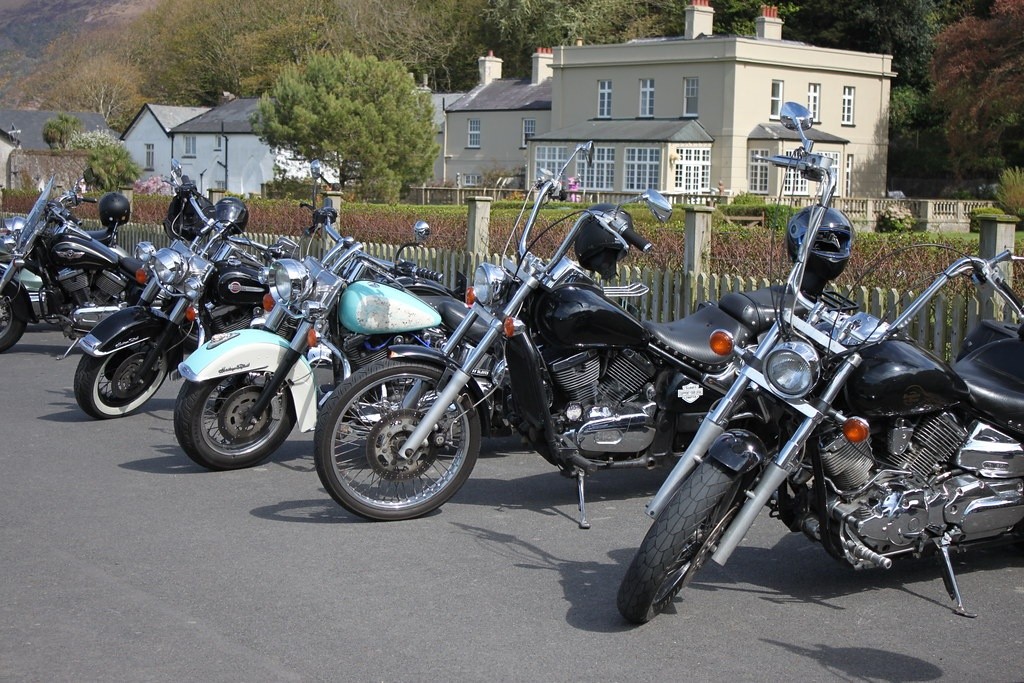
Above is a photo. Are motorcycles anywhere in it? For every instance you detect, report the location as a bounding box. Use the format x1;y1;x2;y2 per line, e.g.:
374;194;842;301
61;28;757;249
1;141;809;526
617;102;1024;627
